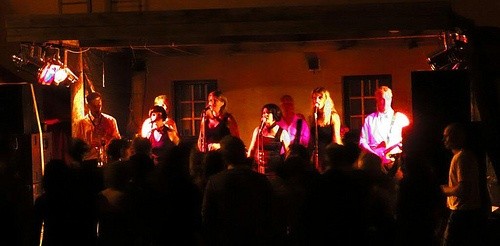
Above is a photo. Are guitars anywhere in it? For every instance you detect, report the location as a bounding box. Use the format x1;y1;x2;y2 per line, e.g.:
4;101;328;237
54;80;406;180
368;138;404;166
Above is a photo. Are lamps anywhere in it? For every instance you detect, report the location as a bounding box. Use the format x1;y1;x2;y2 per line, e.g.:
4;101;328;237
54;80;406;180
9;41;79;89
429;32;482;72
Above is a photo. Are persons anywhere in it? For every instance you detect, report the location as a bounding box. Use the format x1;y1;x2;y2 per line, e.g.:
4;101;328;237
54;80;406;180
438;122;487;246
72;92;121;164
199;134;275;246
247;104;291;176
307;88;344;145
358;86;411;162
197;88;239;153
33;86;500;246
140;95;179;146
270;142;316;190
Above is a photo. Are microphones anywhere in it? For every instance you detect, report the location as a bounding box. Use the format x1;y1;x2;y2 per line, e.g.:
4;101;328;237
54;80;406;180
202;105;211;113
258;118;266;132
314;103;319;117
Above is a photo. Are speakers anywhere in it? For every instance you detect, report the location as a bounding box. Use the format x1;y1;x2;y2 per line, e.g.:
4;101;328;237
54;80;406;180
410;70;471;123
16;132;53;184
19;182;44;204
